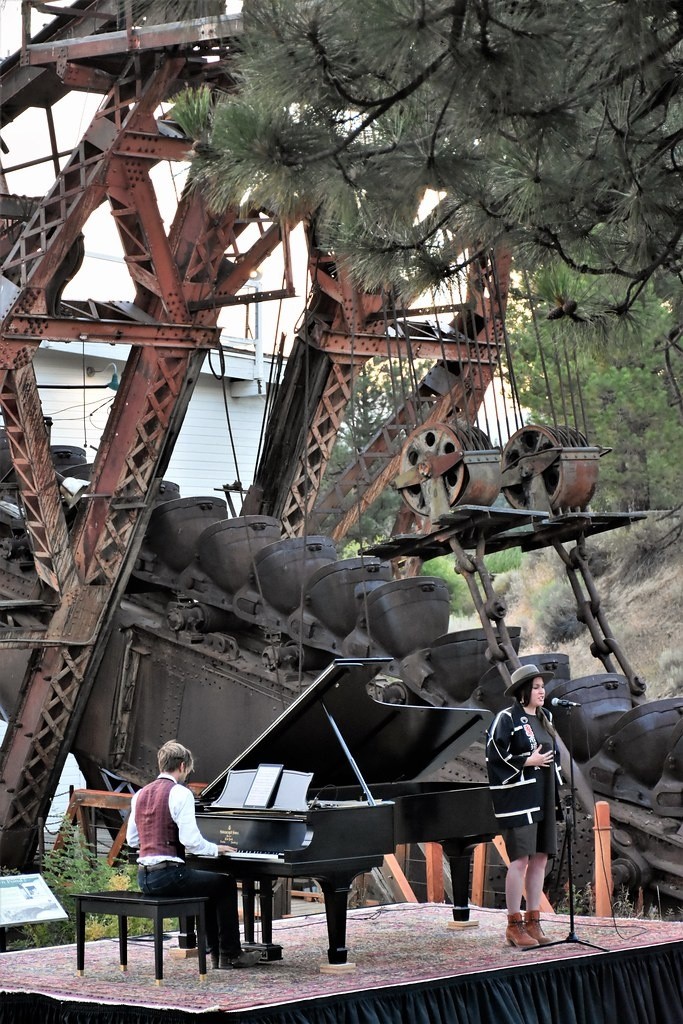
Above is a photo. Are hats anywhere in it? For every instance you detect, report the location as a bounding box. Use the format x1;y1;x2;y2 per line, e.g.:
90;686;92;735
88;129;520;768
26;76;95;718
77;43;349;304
504;664;555;697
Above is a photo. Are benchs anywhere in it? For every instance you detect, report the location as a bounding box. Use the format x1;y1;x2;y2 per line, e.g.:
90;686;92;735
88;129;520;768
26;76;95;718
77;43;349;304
69;889;208;987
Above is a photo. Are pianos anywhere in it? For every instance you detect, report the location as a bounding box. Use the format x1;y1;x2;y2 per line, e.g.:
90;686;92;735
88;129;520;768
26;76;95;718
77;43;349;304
165;659;497;982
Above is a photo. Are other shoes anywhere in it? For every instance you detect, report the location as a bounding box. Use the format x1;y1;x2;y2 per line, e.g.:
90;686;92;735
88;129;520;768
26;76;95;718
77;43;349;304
209;954;219;969
219;951;261;969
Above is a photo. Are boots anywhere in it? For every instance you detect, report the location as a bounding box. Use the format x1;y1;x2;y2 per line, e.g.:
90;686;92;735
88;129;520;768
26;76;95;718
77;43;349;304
505;912;540;948
524;910;552;944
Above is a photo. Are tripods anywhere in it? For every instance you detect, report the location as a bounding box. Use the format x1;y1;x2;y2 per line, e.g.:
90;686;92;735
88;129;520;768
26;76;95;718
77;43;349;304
521;707;610;953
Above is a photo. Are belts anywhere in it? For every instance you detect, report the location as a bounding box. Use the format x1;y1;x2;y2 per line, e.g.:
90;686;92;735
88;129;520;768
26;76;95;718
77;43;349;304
138;862;178;873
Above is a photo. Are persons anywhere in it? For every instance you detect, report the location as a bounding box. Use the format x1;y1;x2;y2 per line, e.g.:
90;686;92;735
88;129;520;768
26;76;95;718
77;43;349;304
126;738;262;969
486;664;564;948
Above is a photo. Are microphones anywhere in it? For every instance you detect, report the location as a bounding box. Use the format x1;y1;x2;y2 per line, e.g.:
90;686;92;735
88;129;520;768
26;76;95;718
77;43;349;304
551;698;582;708
